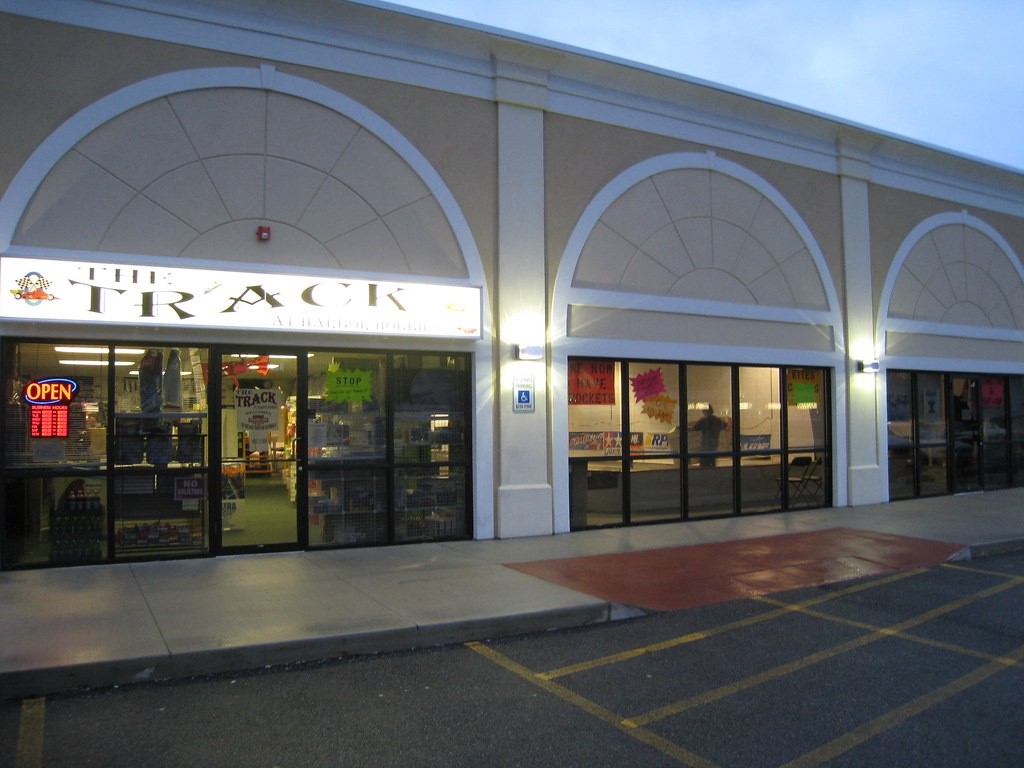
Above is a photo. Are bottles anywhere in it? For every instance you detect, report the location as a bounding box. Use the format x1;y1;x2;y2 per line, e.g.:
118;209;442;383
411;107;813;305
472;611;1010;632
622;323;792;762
49;488;105;547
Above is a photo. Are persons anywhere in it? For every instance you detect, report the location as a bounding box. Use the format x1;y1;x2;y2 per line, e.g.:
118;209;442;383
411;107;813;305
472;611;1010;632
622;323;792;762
676;403;728;468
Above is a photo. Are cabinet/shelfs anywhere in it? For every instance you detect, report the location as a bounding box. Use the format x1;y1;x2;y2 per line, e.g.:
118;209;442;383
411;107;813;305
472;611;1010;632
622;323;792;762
392;406;470;540
307;400;388;544
111;411;208;555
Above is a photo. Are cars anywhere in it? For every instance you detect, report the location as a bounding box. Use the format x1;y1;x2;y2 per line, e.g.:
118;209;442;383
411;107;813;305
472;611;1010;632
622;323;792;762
888;421;1005;471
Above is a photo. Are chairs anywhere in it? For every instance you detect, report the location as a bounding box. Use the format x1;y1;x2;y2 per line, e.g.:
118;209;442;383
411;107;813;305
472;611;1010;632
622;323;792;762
771;456;824;509
